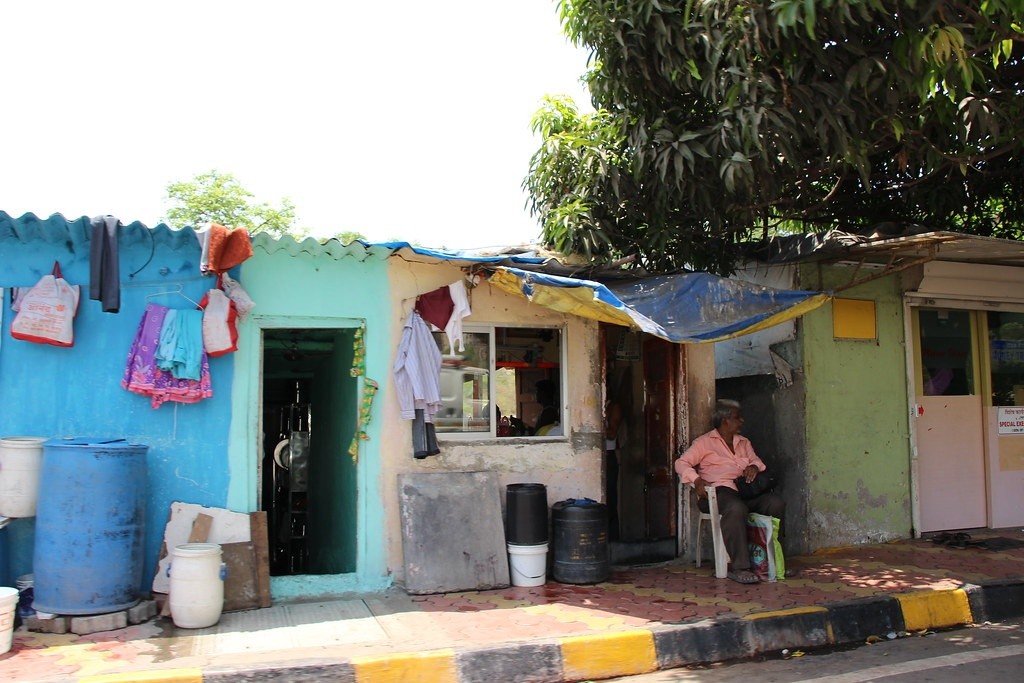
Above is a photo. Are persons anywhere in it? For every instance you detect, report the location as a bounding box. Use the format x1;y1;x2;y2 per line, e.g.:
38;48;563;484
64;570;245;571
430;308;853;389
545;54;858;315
674;398;798;584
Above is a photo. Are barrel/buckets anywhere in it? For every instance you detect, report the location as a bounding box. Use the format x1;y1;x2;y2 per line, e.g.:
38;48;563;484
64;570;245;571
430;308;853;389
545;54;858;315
552;500;610;584
33;435;150;615
16;573;36;618
507;543;548;586
166;543;224;628
506;483;548;545
0;587;20;654
0;437;45;517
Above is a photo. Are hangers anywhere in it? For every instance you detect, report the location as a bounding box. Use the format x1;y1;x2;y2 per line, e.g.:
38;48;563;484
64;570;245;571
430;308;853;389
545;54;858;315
141;282;205;317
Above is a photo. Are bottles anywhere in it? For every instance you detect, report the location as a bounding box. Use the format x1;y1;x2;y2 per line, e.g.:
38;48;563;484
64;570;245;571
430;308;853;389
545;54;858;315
502;416;509;436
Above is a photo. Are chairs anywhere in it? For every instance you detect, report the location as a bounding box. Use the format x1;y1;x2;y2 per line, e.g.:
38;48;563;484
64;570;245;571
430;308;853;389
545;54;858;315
688;482;778;583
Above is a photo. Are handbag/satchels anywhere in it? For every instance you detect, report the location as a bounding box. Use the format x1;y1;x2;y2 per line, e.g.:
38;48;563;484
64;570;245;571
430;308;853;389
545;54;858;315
747;513;786;583
733;471;775;501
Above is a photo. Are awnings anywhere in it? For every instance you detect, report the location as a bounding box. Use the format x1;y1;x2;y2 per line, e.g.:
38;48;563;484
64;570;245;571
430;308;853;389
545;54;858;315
470;261;835;346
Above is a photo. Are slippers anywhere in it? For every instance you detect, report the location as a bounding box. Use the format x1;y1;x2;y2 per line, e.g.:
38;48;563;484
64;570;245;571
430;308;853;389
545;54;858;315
728;569;759;584
785;569;795;578
